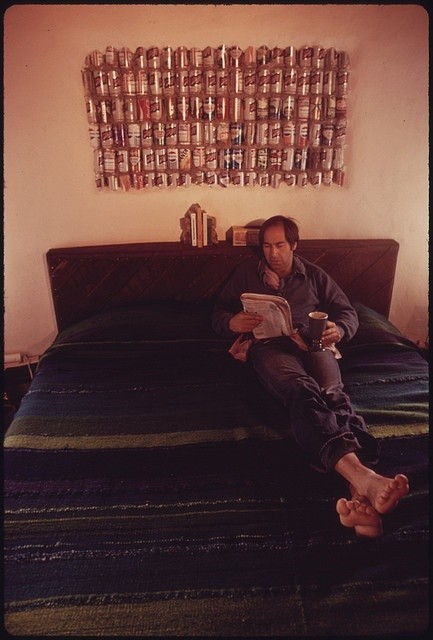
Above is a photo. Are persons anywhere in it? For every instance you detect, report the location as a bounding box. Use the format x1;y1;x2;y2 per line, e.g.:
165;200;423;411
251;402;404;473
209;216;411;539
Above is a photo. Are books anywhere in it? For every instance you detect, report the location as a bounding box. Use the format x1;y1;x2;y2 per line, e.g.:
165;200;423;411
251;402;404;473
240;293;293;339
183;203;219;248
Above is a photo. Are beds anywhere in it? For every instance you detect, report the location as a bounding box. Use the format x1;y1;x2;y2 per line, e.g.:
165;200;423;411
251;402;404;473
0;241;429;635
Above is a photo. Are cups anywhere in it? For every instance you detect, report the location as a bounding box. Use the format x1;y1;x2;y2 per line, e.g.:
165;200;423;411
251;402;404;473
308;312;328;342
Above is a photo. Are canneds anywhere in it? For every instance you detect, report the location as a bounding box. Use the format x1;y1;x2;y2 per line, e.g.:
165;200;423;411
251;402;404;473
311;45;325;69
217;122;229;144
244;172;257;188
230;45;244;67
119;175;131;191
324;47;338;68
206;148;218;170
156;173;167;189
138;99;151;122
133;174;143;190
202;46;217;67
284;46;297;67
103;149;116;173
147;46;160;69
270;174;284;189
153;123;166;145
188;69;203;95
243;98;256;120
309;124;321;147
310;68;323;94
144;173;155;190
218;147;231;171
334;126;346;146
82;70;95;96
216;98;229;120
179;124;191;145
191;122;204;145
310;97;322;121
175;46;189;69
105;175;114;190
124;99;137;122
268;122;281;144
112;124;126;148
190;47;203;68
281;148;294;171
244;69;258;95
322;126;334;146
175;70;189;95
122;71;136;96
337;69;348;92
271;69;283;93
257;45;271;67
323;171;334;187
256;69;270;93
323;95;336;119
111;98;125;123
91;50;105;68
180;173;192;188
195;171;205;186
117;150;128;173
203;70;216;94
332;170;344;187
283;122;295;146
243;46;257;67
167;148;179;169
271;46;284;67
107;70;122;97
230;122;242;146
127;124;140;148
149;70;163;95
94;175;103;191
115;177;122;190
298;46;312;67
294;148;308;171
129;150;141;173
179;149;191;169
297;69;310;95
190;97;203;119
243;149;256;171
269;97;283;120
118;47;132;68
168;172;180;189
232;149;243;170
136;70;148;96
321;148;334;170
134;46;148;68
177;96;190;121
307;147;321;170
230;68;244;95
96;99;112;124
258;148;268;170
93;70;109;96
165;123;178;145
323;71;336;95
105;45;119;68
141;122;154;147
258;174;269;188
284;174;296;187
154;149;167;171
150;99;163;122
336;95;347;113
268;149;283;171
298;97;310;121
162;70;175;94
310;172;322;189
162;45;174;70
94;150;104;174
216;70;229;95
205;171;218;187
338;51;350;69
100;124;114;148
232;172;244;187
256;123;268;145
142;149;155;170
332;148;344;169
202;97;217;121
283;68;298;94
229;98;243;122
296;123;308;146
164;97;177;122
218;172;230;189
217;44;231;68
89;125;101;149
283;96;296;121
297;172;308;189
85;98;97;123
243;122;255;145
257;98;269;121
204;122;217;144
192;146;206;168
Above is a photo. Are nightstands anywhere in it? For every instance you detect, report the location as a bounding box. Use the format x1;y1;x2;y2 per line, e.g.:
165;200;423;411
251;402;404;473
2;361;37;433
